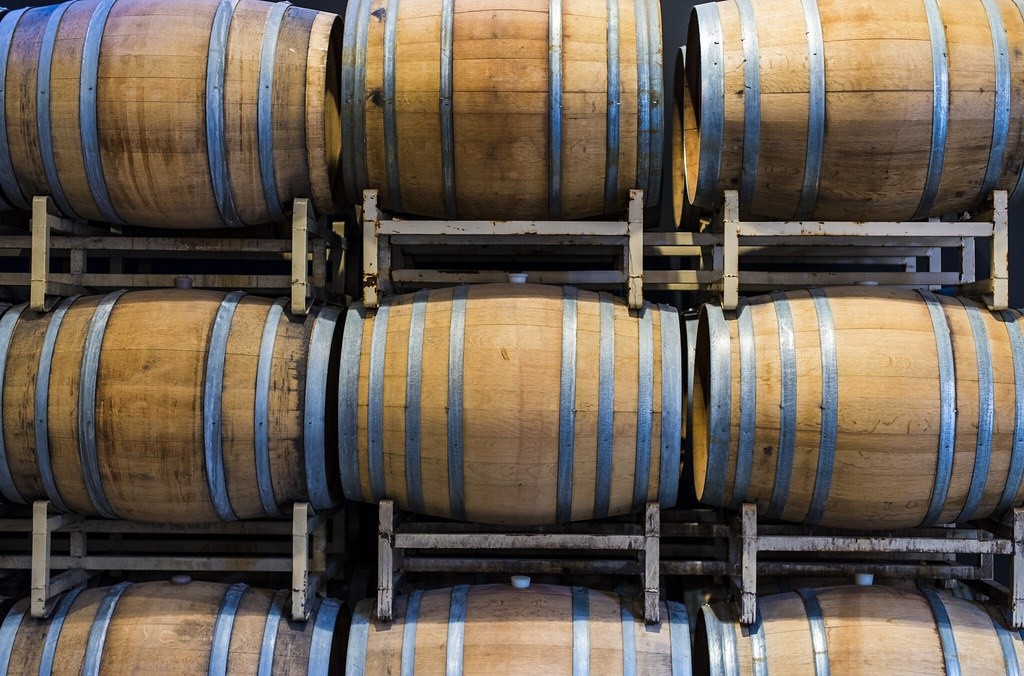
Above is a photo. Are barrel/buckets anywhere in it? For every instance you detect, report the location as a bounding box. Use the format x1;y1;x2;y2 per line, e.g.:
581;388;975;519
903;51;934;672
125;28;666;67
0;1;342;230
678;573;1024;676
1;575;346;676
345;574;693;676
338;271;679;525
343;1;663;229
671;1;1024;234
682;283;1024;530
0;277;344;523
